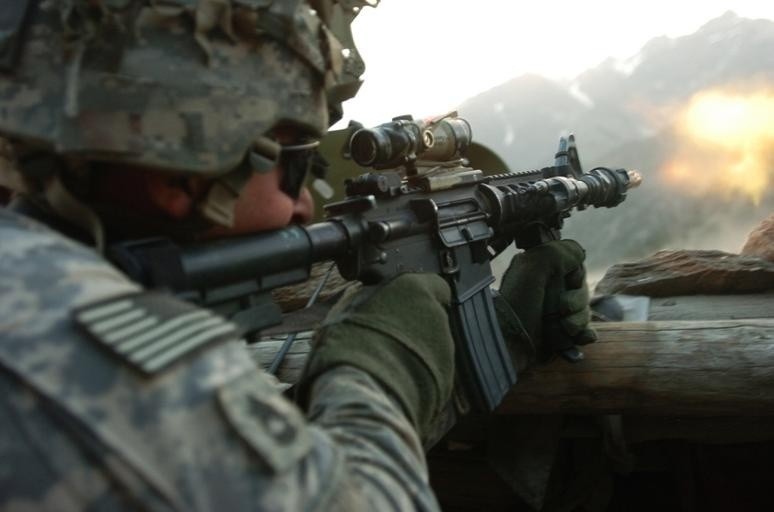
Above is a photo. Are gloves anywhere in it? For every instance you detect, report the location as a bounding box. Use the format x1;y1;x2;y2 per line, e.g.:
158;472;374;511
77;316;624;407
297;274;456;436
498;239;587;357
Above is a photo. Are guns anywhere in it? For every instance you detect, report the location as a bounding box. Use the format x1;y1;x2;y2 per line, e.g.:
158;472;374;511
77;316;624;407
103;112;640;414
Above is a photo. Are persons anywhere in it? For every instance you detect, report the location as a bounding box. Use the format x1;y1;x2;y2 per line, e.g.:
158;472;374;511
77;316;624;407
0;0;601;511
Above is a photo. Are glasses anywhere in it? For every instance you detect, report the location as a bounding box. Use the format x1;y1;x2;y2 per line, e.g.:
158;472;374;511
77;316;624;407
278;141;321;198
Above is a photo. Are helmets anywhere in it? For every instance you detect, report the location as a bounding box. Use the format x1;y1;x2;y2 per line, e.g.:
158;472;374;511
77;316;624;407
0;2;328;176
